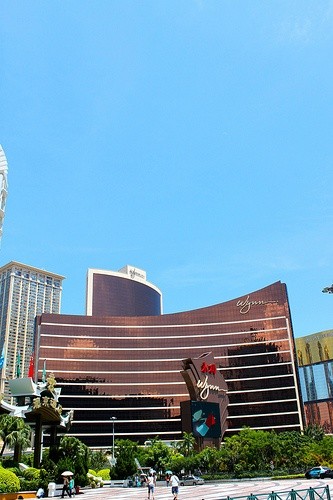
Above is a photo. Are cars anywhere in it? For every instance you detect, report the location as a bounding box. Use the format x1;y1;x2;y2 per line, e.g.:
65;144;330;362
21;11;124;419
305;466;333;480
178;476;204;486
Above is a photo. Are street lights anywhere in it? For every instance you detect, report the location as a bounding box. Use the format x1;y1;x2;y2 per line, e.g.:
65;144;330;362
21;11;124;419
110;416;117;464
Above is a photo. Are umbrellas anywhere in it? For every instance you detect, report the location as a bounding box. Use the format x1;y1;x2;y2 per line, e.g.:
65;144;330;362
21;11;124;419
61;471;74;476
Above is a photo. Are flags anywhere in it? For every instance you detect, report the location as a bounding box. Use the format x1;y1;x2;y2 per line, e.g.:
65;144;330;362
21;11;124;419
0;341;7;370
17;346;21;378
42;360;47;384
28;351;34;378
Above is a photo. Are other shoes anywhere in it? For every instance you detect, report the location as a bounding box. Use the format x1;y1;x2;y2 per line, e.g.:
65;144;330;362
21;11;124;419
173;497;176;500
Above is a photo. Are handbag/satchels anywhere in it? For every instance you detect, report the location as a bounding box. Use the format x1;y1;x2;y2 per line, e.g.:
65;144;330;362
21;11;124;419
71;488;76;493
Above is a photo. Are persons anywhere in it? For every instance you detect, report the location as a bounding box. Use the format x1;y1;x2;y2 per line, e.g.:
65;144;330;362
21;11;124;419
127;471;175;487
60;476;75;499
146;472;156;500
170;472;180;500
36;487;44;499
